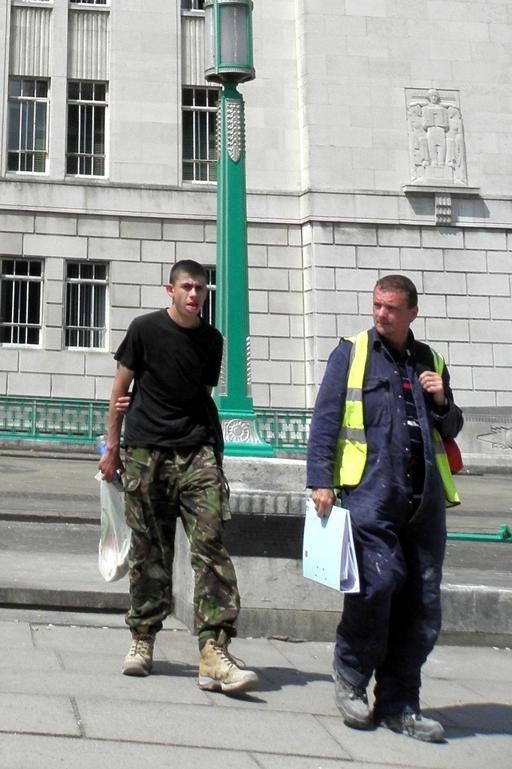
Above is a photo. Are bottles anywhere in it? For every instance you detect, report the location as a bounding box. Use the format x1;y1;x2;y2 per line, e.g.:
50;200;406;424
96;434;124;485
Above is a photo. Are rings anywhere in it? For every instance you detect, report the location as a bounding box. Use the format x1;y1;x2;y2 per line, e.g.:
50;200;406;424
430;381;433;386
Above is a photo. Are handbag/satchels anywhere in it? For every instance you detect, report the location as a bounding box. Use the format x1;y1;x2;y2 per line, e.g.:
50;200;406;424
94;468;133;583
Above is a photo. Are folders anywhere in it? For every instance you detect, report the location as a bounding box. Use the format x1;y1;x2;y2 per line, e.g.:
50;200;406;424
302;498;360;593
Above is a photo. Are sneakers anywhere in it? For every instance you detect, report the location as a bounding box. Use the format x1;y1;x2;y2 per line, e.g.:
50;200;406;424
331;659;371;728
373;700;446;742
199;629;258;694
122;625;157;676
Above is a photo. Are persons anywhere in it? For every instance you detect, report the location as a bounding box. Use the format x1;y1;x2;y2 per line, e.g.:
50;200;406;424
406;89;467;183
305;273;465;742
98;259;259;696
